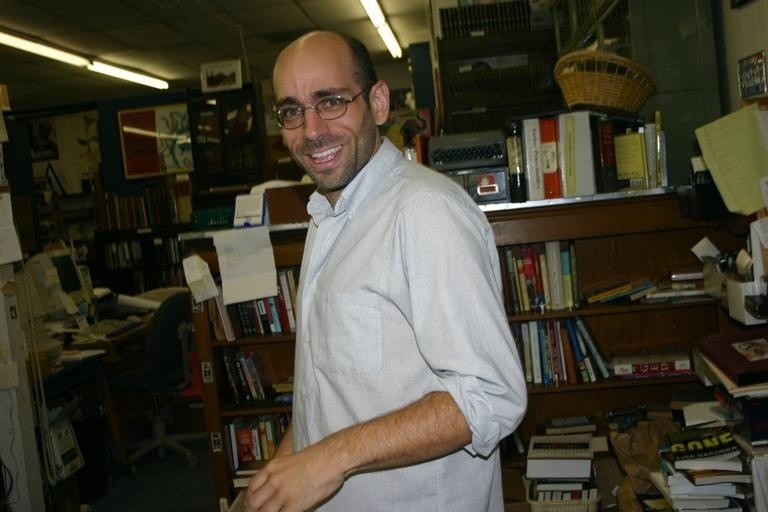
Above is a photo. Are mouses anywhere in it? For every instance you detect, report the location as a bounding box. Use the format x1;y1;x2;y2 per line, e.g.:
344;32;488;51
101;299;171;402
126;314;142;324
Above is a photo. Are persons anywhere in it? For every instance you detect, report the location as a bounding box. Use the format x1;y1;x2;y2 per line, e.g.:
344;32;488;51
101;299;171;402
242;30;532;510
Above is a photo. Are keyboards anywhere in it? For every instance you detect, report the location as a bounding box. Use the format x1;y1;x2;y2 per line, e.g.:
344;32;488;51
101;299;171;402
86;318;147;341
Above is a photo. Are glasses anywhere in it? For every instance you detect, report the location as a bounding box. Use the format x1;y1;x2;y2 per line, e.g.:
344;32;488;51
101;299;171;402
274;86;372;130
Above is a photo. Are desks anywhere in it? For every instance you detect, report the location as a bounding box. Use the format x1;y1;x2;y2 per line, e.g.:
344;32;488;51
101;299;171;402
29;310;154;480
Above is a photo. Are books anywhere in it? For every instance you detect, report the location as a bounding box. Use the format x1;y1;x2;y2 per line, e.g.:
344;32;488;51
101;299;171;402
502;111;647;203
503;240;581;313
513;415;609;510
604;389;768;510
206;262;300;508
33;183;174;294
582;264;705;304
508;316;611;388
606;345;695;380
694;330;768;448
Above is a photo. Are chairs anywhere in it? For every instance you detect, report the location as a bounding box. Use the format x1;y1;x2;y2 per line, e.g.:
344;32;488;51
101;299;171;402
105;286;208;476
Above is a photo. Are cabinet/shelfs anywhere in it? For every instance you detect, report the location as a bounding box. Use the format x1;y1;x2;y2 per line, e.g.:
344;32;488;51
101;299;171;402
1;83;268;295
177;184;768;511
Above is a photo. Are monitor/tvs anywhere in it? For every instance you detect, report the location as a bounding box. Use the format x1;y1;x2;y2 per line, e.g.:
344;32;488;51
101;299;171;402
21;247;89;317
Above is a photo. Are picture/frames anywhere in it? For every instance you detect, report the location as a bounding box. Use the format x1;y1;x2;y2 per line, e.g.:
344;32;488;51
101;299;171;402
735;50;768;102
198;58;242;95
